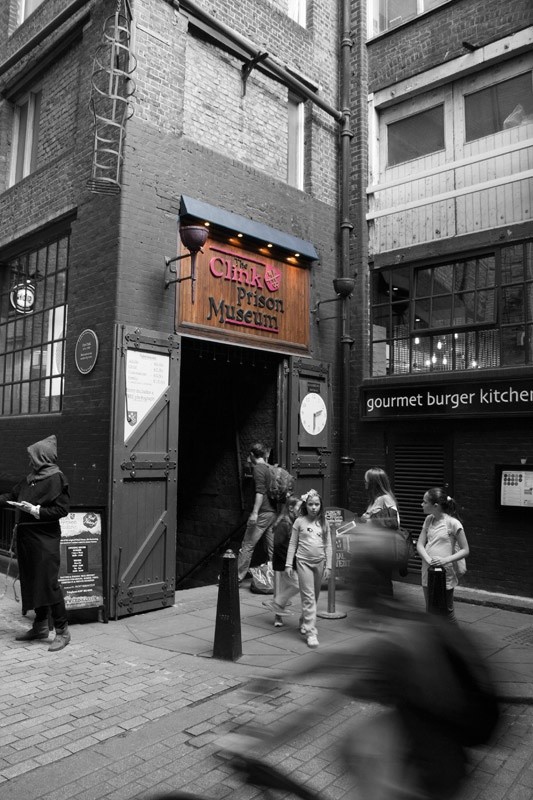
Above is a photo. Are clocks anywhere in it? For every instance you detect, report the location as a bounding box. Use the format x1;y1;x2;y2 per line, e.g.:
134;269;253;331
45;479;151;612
298;393;328;435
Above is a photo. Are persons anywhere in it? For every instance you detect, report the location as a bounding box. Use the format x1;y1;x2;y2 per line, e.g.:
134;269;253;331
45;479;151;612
0;434;72;652
284;489;332;649
272;498;303;627
416;487;470;625
217;443;278;588
359;467;400;528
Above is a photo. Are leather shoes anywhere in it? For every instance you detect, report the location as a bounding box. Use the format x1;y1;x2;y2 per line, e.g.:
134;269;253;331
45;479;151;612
16;627;48;639
49;631;71;650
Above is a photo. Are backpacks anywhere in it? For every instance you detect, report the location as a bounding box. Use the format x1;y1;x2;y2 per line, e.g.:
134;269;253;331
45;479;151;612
260;462;296;503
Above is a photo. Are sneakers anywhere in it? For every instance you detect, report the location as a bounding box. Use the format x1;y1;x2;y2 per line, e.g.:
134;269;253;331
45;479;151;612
309;634;319;646
275;615;283;626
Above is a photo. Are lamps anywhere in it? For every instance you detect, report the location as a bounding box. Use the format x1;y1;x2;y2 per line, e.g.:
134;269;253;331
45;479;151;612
318;277;357;337
164;226;209;304
